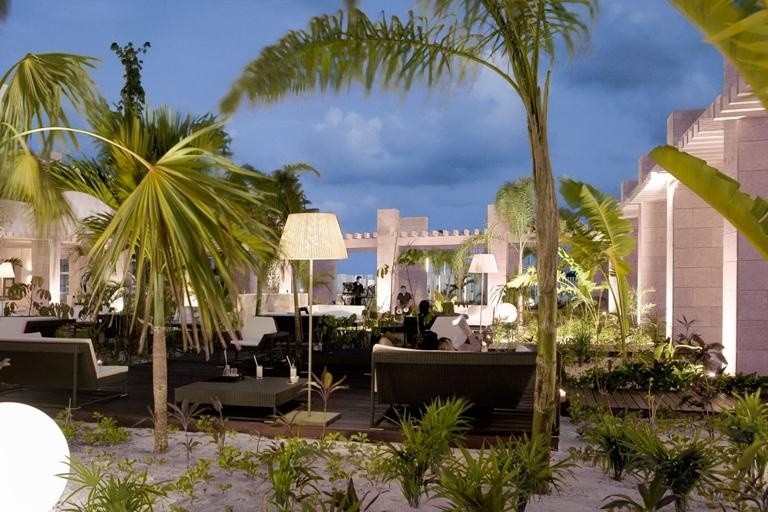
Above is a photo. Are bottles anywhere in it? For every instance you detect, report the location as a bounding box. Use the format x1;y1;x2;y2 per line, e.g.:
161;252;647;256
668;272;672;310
223;365;230;376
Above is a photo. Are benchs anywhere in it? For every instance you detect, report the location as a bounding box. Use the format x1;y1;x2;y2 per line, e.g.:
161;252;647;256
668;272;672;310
1;292;542;429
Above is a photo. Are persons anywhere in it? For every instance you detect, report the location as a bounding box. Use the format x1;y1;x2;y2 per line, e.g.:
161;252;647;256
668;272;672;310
397;285;413;314
353;276;365;304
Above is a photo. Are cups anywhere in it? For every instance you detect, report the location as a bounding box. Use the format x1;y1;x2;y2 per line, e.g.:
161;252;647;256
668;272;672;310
230;368;238;375
256;365;264;381
290;366;299;384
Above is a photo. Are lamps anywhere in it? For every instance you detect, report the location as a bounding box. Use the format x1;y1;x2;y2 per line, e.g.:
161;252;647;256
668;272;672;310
463;251;500;336
0;260;17;295
271;212;351;429
